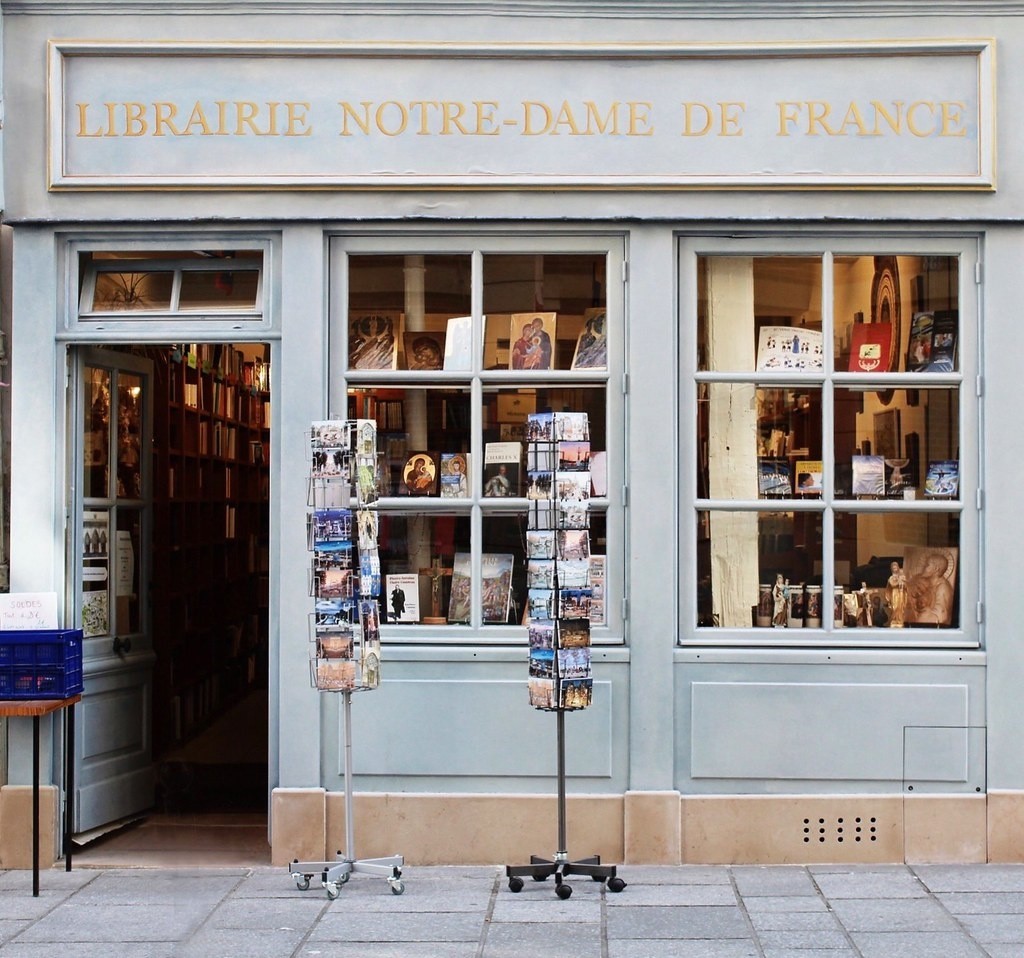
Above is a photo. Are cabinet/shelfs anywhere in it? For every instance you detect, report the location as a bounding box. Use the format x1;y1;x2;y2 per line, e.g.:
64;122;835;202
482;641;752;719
743;356;862;641
92;343;550;754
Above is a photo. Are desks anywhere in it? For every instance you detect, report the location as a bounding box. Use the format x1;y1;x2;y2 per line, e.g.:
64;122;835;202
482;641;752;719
0;690;87;900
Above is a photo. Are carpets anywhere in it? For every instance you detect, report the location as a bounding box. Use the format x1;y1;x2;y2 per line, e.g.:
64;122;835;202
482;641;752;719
153;760;269;814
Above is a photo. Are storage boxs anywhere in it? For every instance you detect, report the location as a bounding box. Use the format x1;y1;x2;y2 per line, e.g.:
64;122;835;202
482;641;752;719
0;629;84;701
0;782;63;876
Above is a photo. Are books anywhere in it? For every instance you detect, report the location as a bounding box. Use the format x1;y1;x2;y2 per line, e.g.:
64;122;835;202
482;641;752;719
852;456;959;497
528;411;593;708
849;322;892;372
508;313;557;369
168;343;272;740
767;430;809;457
759;461;823;494
349;396;408;556
448;552;514;623
571;308;607;370
697;364;709;538
908;309;958;365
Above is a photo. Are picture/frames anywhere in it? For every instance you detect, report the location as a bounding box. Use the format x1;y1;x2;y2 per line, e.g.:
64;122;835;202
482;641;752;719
568;308;607;371
445;548;515;625
396;450;444;500
506;312;555;375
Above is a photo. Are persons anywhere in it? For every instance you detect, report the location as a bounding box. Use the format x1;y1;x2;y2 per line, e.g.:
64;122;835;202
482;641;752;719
772;574;789;625
798;473;814;488
885;562;908;628
486;465;508;496
909;554;953;622
406;458;432;491
852;582;872;627
309;419;381;691
391;585;406;620
512;318;552;370
